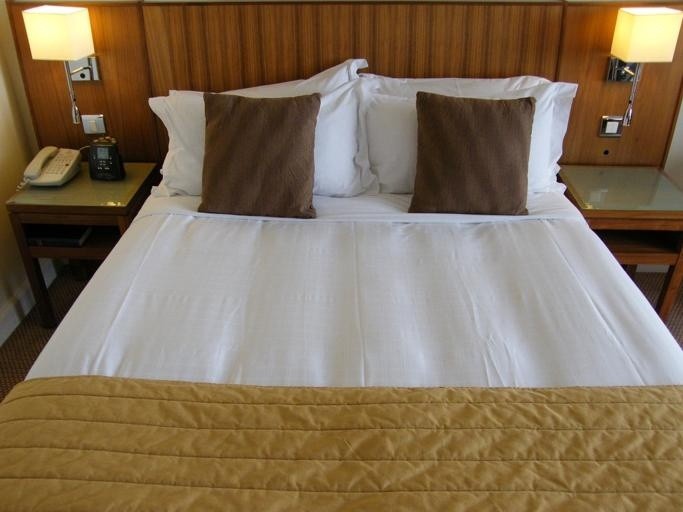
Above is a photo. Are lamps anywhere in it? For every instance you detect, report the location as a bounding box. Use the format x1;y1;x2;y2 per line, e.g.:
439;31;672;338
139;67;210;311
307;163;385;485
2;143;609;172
606;6;683;127
22;4;101;125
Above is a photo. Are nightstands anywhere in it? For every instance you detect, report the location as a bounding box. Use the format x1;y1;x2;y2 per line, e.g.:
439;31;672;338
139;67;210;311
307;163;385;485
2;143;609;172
6;162;159;331
557;164;683;323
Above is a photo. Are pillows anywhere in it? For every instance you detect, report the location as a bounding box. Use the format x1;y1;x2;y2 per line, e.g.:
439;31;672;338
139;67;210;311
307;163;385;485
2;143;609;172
149;77;377;198
365;82;579;198
359;72;551;97
407;91;535;216
169;59;369;99
197;92;321;219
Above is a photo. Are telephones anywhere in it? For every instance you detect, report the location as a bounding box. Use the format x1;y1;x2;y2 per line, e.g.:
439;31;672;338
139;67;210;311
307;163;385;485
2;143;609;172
23;146;81;186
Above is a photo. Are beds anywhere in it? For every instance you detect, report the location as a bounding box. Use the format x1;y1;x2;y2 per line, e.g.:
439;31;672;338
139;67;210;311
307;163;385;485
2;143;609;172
0;0;683;512
